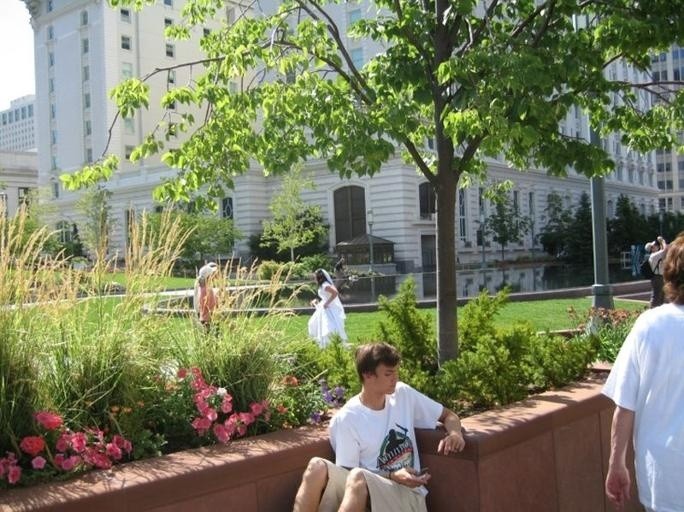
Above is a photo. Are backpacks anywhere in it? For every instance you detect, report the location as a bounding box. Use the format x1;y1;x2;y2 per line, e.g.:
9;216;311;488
639;257;661;280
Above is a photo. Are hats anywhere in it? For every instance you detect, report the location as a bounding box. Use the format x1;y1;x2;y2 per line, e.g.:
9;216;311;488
197;265;216;281
645;240;656;253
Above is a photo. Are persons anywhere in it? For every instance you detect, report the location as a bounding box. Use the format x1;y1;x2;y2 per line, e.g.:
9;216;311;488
198;266;218;331
644;236;668;309
292;341;465;512
307;269;348;350
334;258;346;278
193;262;217;319
601;235;684;512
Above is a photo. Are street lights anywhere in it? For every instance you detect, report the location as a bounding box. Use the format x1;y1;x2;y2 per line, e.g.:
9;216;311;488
367;209;376;303
480;214;487;290
659;212;664;236
571;9;625;339
